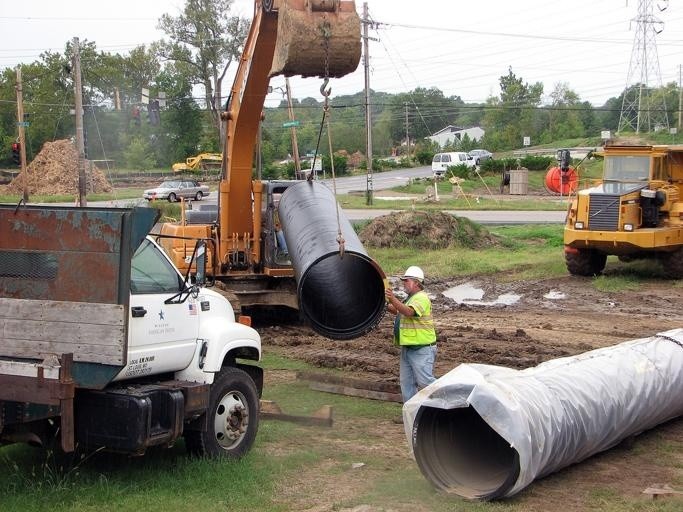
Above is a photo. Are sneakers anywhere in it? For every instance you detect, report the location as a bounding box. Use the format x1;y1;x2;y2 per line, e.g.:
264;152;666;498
394;416;403;423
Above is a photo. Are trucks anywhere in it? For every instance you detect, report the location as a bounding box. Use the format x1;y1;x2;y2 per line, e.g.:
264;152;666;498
1;194;268;471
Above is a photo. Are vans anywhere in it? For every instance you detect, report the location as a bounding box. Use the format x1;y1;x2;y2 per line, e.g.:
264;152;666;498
431;151;476;177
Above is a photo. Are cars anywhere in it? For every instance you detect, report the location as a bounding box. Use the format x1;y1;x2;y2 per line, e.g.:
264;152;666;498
141;178;210;203
468;148;493;165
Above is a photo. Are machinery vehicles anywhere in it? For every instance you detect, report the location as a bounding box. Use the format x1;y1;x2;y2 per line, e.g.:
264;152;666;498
562;140;682;279
145;0;363;332
171;150;224;175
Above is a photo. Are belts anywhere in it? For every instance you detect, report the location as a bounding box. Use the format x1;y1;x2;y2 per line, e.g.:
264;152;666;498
423;341;436;346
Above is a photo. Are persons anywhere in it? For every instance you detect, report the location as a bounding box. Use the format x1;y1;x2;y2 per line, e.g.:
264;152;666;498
384;265;438;424
270;204;288;258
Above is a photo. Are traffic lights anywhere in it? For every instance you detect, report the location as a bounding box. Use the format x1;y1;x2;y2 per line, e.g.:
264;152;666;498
12;143;19;165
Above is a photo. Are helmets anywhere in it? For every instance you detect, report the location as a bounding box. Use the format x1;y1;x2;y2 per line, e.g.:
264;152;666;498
400;266;425;282
